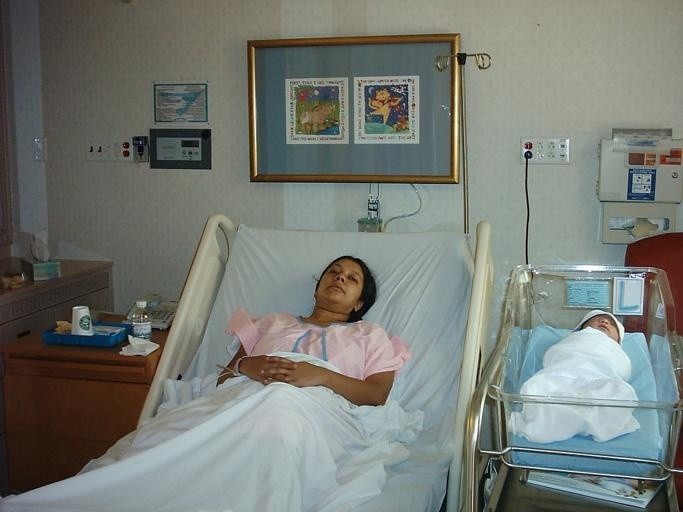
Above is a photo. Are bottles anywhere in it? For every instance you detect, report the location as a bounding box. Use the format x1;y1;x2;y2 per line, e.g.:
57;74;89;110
130;299;152;344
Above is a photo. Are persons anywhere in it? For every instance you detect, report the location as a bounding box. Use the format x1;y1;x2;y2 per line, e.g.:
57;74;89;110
511;309;640;445
215;255;396;406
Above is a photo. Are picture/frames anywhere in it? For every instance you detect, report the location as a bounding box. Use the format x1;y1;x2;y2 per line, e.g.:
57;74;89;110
247;33;463;184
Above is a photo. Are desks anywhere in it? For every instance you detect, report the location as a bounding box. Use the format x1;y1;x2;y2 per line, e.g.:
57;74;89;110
1;258;114;349
2;313;171;497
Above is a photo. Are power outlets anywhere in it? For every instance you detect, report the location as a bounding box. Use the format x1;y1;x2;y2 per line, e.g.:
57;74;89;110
519;136;570;166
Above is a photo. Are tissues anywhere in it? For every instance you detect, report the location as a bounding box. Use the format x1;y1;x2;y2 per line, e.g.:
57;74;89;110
19;240;62;281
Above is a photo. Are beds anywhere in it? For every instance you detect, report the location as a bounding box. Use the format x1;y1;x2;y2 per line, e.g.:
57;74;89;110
462;263;683;512
136;214;493;511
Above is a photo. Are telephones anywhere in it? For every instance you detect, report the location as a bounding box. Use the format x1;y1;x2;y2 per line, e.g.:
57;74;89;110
122;293;178;330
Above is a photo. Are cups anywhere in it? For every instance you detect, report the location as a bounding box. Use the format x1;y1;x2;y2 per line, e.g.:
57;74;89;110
70;304;93;337
